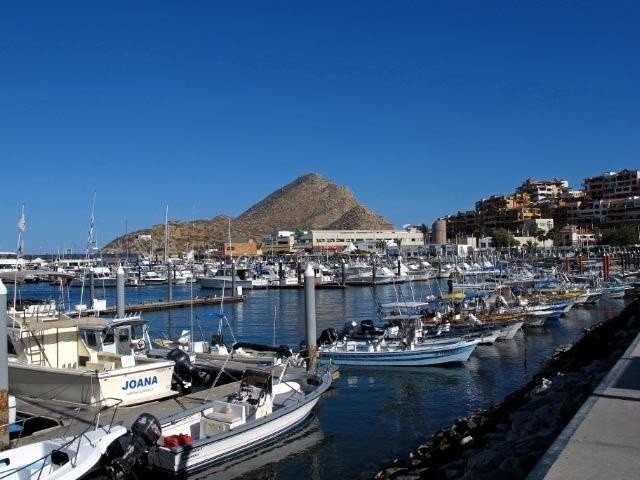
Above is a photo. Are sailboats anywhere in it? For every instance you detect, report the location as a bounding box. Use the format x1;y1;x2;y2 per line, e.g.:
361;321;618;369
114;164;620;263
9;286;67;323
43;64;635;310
612;299;624;306
381;257;523;338
557;317;577;330
514;332;525;345
564;309;592;323
525;327;554;343
470;344;501;359
494;338;520;357
338;365;473;389
419;260;525;340
574;250;613;308
544;318;568;335
613;250;640;298
340;252;502;344
497;259;545;326
300;252;483;365
152;243;307;368
545;250;574;317
463;356;481;372
71;190;196;366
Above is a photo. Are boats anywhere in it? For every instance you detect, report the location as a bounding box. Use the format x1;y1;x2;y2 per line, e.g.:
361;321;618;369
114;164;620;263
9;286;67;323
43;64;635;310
187;415;324;480
0;191;217;287
148;344;338;474
134;355;334;479
130;268;291;381
50;191;136;287
0;204;76;285
198;220;494;289
